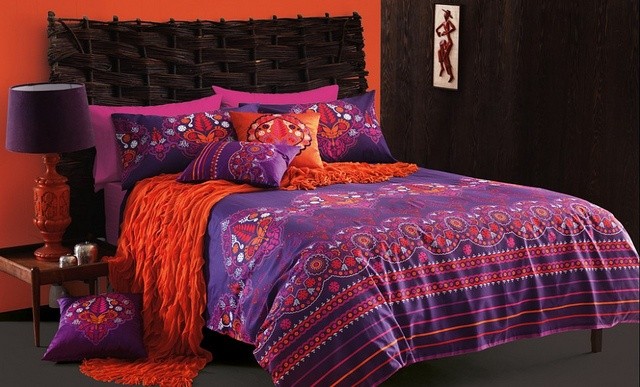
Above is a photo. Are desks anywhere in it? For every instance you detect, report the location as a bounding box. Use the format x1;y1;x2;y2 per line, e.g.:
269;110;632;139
1;240;118;348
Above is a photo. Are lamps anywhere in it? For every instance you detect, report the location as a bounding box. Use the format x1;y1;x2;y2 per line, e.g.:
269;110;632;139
6;79;98;263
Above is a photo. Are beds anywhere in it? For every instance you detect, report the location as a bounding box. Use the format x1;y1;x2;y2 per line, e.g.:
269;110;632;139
47;9;638;385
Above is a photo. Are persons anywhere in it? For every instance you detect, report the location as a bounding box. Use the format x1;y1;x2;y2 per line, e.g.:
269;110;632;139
435;7;456;82
438;39;446;76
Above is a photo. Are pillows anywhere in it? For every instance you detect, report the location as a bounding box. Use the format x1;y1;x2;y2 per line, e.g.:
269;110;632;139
210;82;339;105
109;107;240;194
85;91;226;194
228;107;326;170
238;87;398;165
40;291;150;361
177;138;302;186
